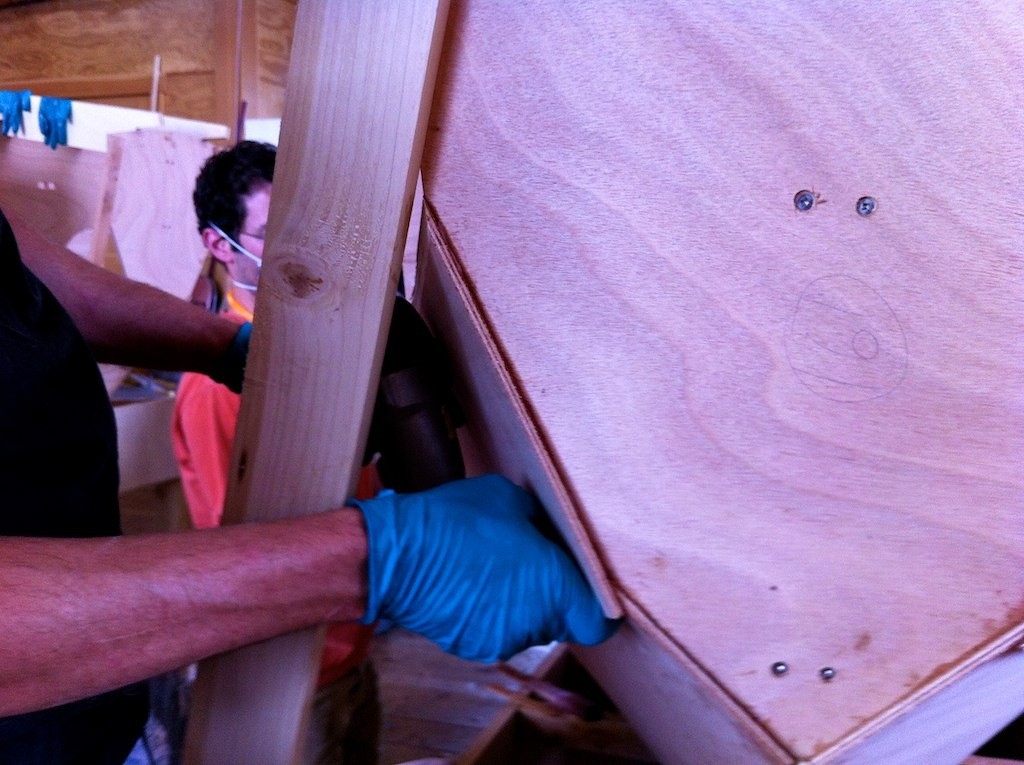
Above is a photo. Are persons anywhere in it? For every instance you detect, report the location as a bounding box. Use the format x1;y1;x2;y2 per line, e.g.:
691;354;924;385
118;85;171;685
167;138;384;765
0;207;623;765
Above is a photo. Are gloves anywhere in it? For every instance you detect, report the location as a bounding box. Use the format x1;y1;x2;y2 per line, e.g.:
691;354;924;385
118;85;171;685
342;475;618;666
